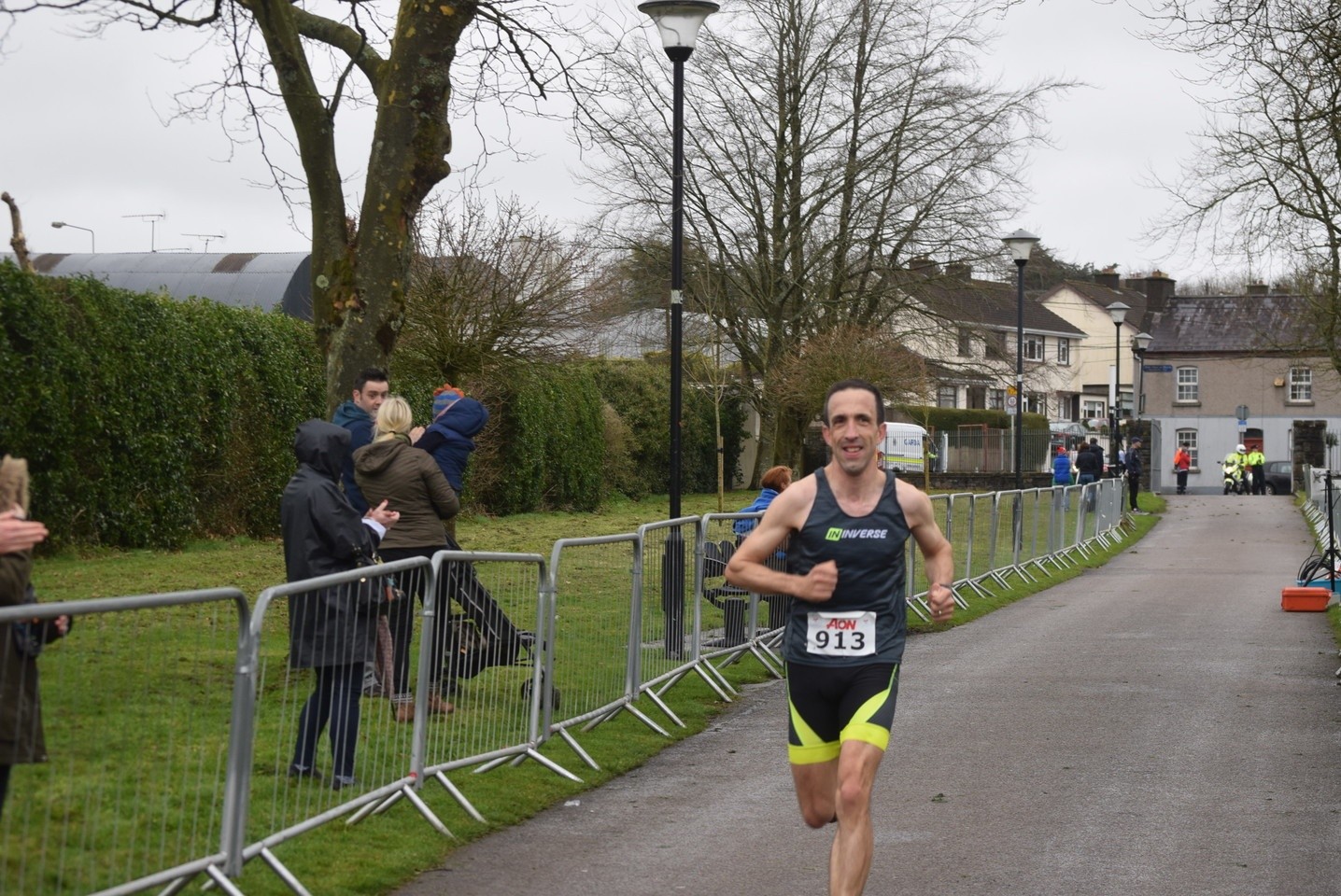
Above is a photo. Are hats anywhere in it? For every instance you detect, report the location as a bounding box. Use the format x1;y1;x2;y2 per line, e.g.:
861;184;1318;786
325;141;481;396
1131;437;1142;443
1058;447;1066;454
1090;438;1097;442
1251;444;1258;449
1183;442;1189;446
432;390;460;420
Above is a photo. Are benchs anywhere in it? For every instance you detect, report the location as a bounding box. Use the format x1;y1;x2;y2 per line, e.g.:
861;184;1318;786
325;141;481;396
703;540;772;647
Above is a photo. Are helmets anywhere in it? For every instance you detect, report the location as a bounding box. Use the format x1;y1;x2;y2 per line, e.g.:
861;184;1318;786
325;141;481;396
1236;444;1246;454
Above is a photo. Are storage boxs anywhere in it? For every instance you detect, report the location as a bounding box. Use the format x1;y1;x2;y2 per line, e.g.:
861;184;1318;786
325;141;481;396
1281;579;1341;610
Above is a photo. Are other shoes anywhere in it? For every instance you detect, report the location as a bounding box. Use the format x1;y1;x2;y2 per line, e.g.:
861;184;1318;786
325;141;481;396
430;679;453;691
428;695;453;715
394;687;412;698
289;763;325;780
1087;500;1091;512
364;683;387;697
394;703;415;722
333;777;358;790
1065;508;1068;511
1132;508;1150;515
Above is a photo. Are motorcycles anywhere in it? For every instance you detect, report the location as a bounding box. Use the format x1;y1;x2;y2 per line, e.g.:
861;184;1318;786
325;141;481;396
1216;458;1244;495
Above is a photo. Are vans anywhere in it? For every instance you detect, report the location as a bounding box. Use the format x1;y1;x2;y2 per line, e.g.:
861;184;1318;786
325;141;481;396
875;421;940;475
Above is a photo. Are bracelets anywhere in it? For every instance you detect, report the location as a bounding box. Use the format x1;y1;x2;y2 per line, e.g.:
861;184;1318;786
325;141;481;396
940;583;952;589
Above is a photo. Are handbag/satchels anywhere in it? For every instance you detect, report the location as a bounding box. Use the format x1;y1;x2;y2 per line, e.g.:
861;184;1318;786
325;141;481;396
355;555;407;616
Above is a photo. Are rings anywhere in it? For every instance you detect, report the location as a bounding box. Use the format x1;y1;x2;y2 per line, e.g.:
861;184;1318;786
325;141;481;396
938;610;942;616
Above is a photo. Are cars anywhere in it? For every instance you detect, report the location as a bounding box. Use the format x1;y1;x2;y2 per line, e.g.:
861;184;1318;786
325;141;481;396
1244;460;1292;495
1047;417;1109;454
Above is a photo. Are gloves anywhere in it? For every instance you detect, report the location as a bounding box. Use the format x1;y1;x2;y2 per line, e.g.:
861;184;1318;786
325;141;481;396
434;384;464;397
1174;464;1178;470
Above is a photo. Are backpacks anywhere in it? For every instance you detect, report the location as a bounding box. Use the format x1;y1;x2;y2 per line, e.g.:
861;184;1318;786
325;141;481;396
733;505;787;559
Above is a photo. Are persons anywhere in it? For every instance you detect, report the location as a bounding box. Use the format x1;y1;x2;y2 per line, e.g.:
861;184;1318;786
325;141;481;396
1053;446;1071;512
279;366;489;789
1126;435;1145;513
1248;444;1267;495
1229;444;1254;495
732;465;795;630
1075;442;1097;513
1089;437;1103;481
1173;442;1191;494
0;448;73;812
723;380;957;896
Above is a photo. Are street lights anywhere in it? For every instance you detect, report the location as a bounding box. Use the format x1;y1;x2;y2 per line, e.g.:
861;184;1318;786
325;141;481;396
1000;228;1041;550
1134;332;1153;416
1105;300;1131;478
50;221;97;253
637;0;725;664
